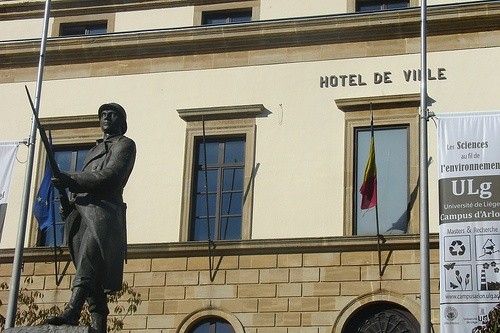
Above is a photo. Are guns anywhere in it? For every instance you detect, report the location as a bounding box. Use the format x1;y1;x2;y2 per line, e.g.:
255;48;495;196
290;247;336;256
25;84;72;219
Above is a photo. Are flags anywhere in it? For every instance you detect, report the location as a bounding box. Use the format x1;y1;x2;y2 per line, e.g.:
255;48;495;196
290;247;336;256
357;134;376;208
32;138;64;231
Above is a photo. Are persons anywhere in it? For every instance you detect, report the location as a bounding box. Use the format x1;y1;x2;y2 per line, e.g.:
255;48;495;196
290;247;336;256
44;103;136;333
